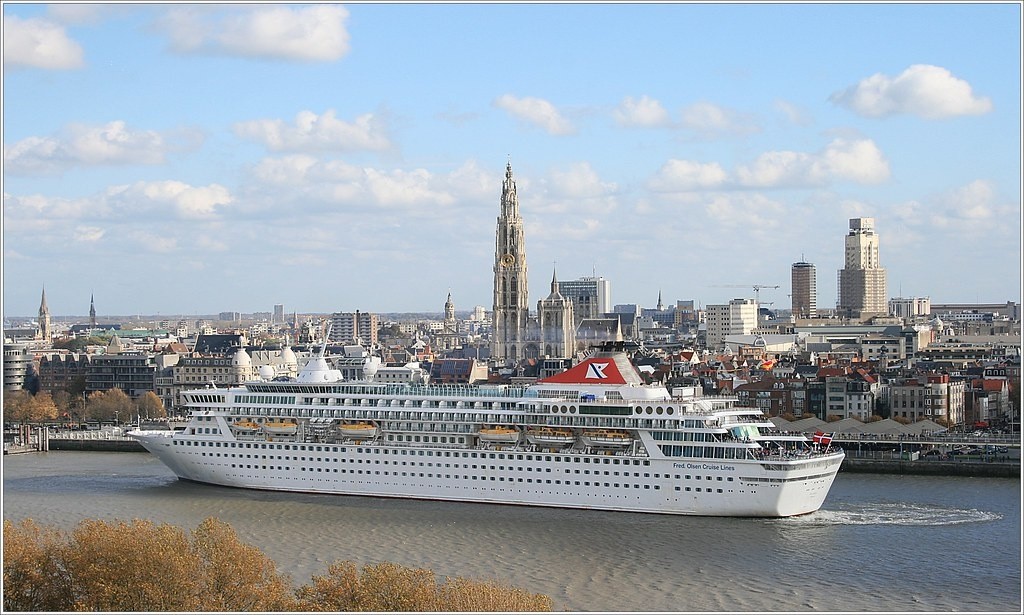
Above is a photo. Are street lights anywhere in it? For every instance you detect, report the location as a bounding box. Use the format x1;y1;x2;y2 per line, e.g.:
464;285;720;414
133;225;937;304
83;391;85;421
1008;402;1014;438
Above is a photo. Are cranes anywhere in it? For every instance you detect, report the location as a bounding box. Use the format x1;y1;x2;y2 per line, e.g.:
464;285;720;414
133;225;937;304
709;283;779;310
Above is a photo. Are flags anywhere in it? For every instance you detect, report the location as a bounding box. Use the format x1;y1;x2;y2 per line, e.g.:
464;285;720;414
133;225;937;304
813;431;833;445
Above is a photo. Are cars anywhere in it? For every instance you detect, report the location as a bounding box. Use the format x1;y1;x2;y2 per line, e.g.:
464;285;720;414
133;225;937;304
922;418;1010;459
4;423;78;432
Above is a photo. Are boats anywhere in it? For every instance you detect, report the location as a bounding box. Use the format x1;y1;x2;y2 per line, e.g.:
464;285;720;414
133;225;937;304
125;321;847;517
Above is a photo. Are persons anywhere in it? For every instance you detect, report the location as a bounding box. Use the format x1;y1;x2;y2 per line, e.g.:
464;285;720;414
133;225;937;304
724;434;751;444
772;428;806;436
838;431;916;441
755;445;842;457
